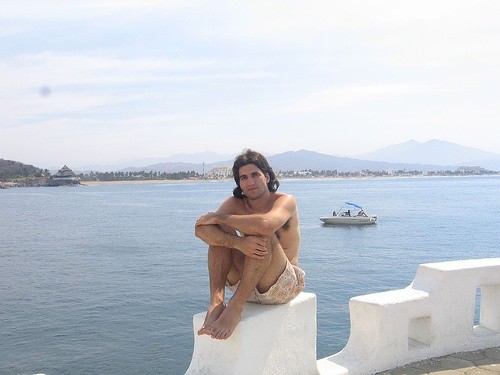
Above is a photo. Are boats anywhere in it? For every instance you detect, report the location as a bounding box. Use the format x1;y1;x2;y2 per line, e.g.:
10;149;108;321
318;200;377;225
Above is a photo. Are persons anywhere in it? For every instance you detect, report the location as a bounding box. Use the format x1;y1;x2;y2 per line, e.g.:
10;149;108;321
358;210;368;217
195;148;304;340
